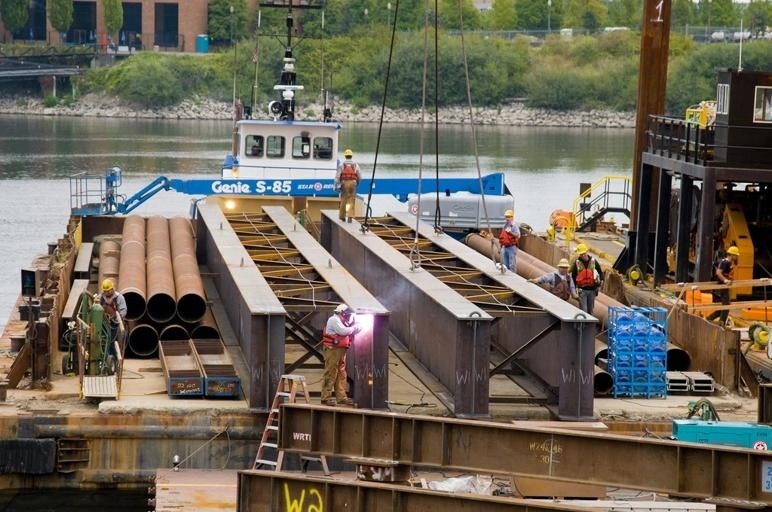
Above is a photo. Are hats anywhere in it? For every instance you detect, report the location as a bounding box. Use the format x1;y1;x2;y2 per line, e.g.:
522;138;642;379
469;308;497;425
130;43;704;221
556;258;572;268
334;304;349;314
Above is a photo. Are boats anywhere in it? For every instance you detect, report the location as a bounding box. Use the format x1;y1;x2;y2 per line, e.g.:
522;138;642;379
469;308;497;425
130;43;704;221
59;1;515;245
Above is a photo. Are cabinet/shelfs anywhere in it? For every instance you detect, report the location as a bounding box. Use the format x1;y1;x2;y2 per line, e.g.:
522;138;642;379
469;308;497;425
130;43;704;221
608;306;668;400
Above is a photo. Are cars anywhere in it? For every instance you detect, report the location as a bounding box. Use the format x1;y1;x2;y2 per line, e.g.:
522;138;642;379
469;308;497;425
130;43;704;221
527;36;544;47
694;27;771;46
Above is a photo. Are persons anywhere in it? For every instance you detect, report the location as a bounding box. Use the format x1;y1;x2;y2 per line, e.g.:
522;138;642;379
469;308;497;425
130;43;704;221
706;246;740;327
498;210;521;274
98;279;128;357
321;304;362;405
334;149;360;223
567;244;604;314
528;258;580;309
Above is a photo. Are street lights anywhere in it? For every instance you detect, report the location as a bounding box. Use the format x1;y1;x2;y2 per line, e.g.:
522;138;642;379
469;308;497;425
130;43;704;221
546;1;553;32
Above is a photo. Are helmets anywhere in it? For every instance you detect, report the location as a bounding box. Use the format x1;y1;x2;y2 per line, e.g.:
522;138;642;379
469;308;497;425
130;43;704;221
344;147;354;157
575;241;590;255
100;279;116;291
503;209;514;217
727;246;742;257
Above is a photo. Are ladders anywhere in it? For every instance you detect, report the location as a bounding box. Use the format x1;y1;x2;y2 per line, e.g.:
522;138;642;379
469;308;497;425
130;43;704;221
252;375;331;476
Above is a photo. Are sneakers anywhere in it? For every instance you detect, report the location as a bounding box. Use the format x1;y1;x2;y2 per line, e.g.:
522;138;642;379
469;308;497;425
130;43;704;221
321;397;335;406
338;396;354;406
